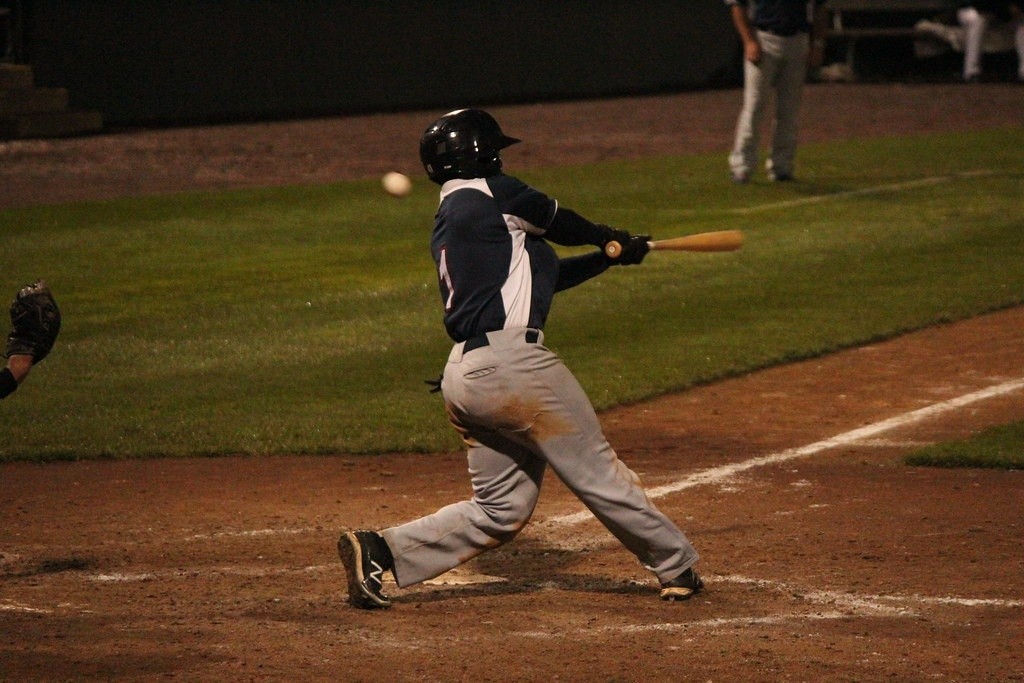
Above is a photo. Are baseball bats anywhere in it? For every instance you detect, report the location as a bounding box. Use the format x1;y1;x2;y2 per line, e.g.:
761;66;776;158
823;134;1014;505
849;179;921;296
603;224;748;259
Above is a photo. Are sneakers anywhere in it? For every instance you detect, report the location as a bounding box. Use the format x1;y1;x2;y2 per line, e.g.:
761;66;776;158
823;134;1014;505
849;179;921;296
659;567;706;600
336;529;393;609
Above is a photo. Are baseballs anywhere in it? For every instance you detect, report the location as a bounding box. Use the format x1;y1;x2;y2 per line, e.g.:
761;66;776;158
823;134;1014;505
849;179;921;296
380;169;414;197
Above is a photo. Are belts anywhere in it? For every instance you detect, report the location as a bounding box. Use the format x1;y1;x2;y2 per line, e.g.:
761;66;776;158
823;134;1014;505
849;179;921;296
755;26;805;38
463;330;539;356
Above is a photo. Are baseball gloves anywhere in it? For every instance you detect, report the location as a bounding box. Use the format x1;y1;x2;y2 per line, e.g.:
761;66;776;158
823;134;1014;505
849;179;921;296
0;277;62;363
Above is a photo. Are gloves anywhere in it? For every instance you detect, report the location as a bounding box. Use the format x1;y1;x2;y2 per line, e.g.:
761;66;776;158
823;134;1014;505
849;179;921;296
617;235;653;265
595;225;631;250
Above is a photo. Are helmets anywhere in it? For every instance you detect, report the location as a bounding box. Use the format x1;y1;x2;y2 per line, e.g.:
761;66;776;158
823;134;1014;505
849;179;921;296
418;108;522;184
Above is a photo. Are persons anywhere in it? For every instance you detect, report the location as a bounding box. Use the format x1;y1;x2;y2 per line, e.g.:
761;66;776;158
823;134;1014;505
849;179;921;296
339;109;703;608
724;0;1024;184
0;281;61;401
